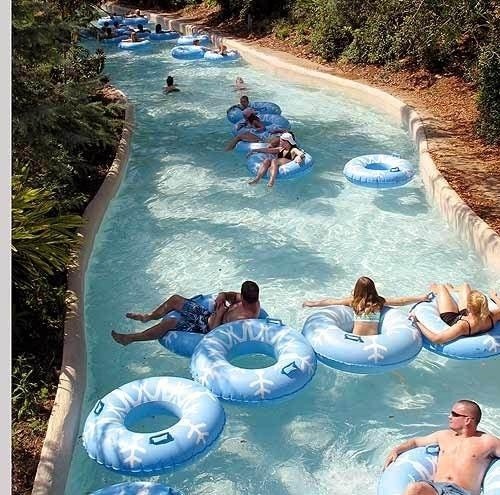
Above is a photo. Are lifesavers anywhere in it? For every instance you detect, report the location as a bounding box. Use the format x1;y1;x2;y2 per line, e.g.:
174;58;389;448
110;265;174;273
136;30;151;37
226;102;281;123
171;45;203;59
237;138;300;153
189;319;317;403
81;376;226;476
112;24;128;32
125;14;148;24
204;48;240;61
376;445;499;494
302;304;423;374
232;114;290;142
409;288;500;360
149;31;179;40
246;149;314;180
343;154;415;188
98;17;119;24
118;39;152;50
176;35;210;45
158;291;270;357
100;33;128;46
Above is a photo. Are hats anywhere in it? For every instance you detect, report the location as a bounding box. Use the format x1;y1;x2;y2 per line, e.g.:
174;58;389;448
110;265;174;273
242;108;258;117
280;132;296;145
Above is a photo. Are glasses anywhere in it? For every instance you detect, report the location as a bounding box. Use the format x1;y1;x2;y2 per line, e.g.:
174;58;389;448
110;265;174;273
452;411;468;417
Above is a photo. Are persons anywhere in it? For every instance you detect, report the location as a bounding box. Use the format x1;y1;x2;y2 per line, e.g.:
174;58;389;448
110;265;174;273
236;108;266;133
111;281;260;347
302;277;428;337
162;75;180;94
122;9;146;19
120;31;145;42
127;24;152;33
155;24;177;34
238;96;249;109
381;400;500;495
226;130;296;153
215;45;237;56
408;281;500;349
193;39;212;53
245;133;305;188
91;14;121;40
228;76;251;94
